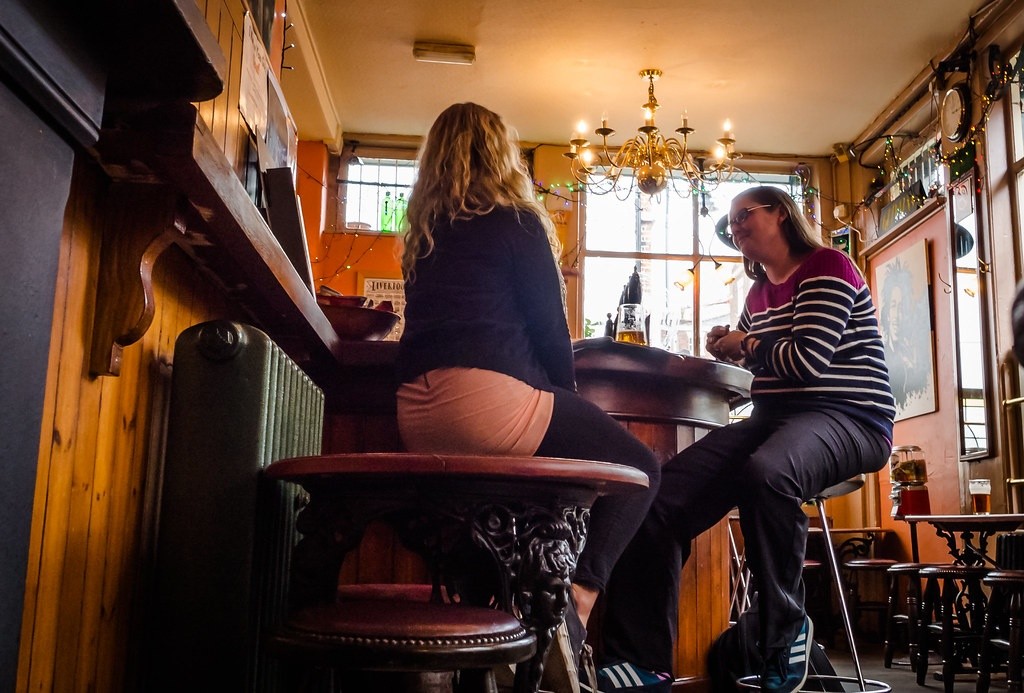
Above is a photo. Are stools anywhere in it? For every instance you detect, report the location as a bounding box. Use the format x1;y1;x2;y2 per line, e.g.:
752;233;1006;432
265;448;651;692
729;474;893;693
798;558;1024;693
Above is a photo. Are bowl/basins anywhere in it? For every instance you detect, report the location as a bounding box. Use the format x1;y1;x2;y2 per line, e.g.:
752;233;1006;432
316;283;402;341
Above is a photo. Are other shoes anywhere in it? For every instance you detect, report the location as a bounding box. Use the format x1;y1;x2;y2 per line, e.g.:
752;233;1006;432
493;664;516;688
544;590;598;693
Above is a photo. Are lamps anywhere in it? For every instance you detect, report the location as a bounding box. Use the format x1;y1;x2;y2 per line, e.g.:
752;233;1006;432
940;85;971;143
562;69;744;205
412;41;476;64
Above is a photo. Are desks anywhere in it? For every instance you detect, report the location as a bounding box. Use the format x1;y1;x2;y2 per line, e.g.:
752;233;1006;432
905;513;1024;682
86;105;339;378
808;526;894;650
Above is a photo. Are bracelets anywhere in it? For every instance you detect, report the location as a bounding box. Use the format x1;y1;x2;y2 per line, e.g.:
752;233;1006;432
740;335;759;359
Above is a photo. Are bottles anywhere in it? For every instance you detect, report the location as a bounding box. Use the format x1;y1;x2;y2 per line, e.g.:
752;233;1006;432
395;193;409;233
380;191;394;232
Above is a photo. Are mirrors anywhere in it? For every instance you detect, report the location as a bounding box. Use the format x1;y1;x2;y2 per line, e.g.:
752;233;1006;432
945;167;994;462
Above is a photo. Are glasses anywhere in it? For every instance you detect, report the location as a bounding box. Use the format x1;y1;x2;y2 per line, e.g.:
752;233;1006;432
725;205;772;234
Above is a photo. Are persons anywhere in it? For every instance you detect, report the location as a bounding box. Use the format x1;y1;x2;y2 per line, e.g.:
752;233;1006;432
577;185;894;693
393;101;661;693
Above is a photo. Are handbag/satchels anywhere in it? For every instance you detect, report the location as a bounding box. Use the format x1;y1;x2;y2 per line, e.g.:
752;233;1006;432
708;592;846;693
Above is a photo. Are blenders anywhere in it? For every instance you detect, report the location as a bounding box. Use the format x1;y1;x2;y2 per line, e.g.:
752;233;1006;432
888;445;930;519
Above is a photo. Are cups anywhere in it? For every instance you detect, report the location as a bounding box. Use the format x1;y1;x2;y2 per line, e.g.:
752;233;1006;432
969;479;991;515
616;303;648;346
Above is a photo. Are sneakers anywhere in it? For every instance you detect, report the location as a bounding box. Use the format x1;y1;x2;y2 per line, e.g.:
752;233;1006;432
762;615;814;693
597;661;674;693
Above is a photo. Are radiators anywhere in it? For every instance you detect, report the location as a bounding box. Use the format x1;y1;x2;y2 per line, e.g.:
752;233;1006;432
996;533;1024;659
132;318;325;693
